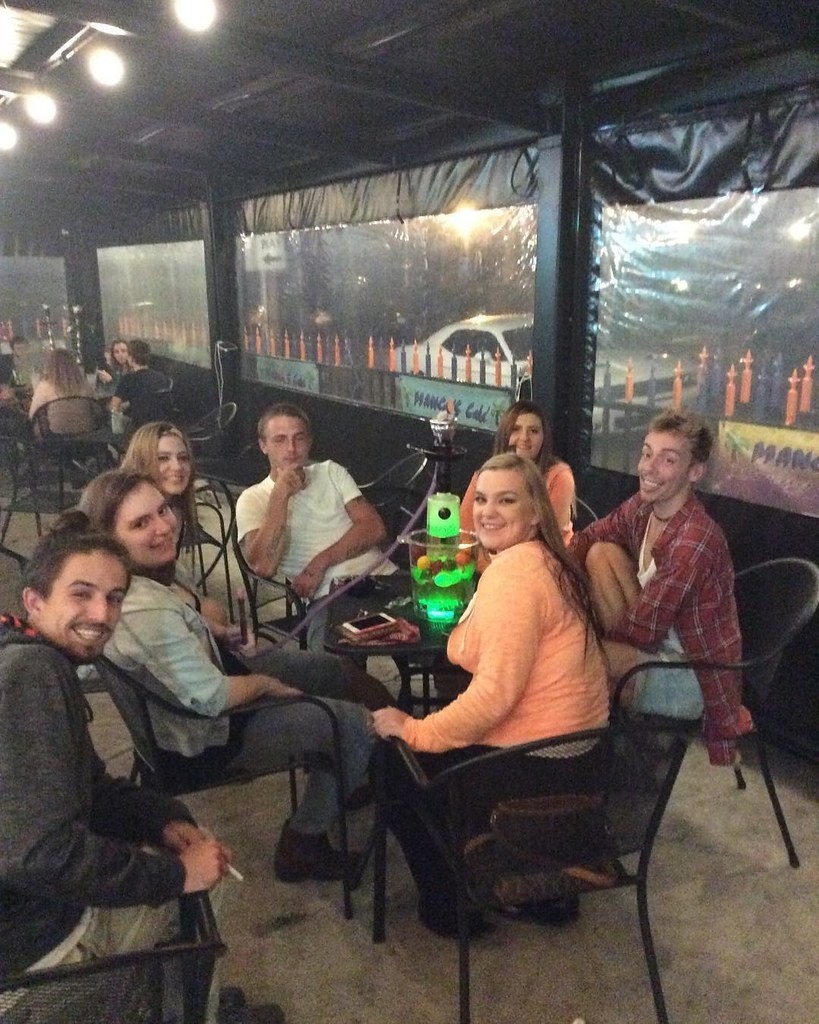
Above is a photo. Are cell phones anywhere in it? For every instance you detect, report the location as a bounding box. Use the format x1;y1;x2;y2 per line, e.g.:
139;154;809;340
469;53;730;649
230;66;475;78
342;612;397;635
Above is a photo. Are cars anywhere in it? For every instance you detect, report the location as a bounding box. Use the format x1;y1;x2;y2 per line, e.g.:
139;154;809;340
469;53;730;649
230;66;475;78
395;314;703;439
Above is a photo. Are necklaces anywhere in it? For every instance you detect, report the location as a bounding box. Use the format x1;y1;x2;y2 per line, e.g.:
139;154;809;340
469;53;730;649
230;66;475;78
651;504;673;526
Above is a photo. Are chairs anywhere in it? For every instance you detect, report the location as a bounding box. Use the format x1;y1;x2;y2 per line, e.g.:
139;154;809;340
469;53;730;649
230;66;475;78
0;376;819;1024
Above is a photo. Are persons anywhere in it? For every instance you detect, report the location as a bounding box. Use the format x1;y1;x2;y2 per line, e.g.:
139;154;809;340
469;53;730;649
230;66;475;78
0;398;743;1024
0;339;171;480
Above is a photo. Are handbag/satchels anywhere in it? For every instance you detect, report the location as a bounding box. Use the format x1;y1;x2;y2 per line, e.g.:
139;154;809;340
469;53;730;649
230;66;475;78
491;784;621;906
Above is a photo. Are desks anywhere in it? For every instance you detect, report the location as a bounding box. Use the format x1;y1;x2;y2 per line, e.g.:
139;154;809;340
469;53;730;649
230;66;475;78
321;573;479;945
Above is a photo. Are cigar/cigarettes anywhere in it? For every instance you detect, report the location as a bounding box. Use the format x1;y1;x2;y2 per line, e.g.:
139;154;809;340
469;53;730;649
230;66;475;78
227;863;243;883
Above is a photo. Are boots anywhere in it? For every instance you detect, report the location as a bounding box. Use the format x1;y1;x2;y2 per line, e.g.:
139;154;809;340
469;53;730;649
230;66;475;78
274;819;367;880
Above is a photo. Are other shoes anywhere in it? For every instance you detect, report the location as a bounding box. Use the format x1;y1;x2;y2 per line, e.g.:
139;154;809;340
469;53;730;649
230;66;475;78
421;905;483;934
218;988;286;1024
